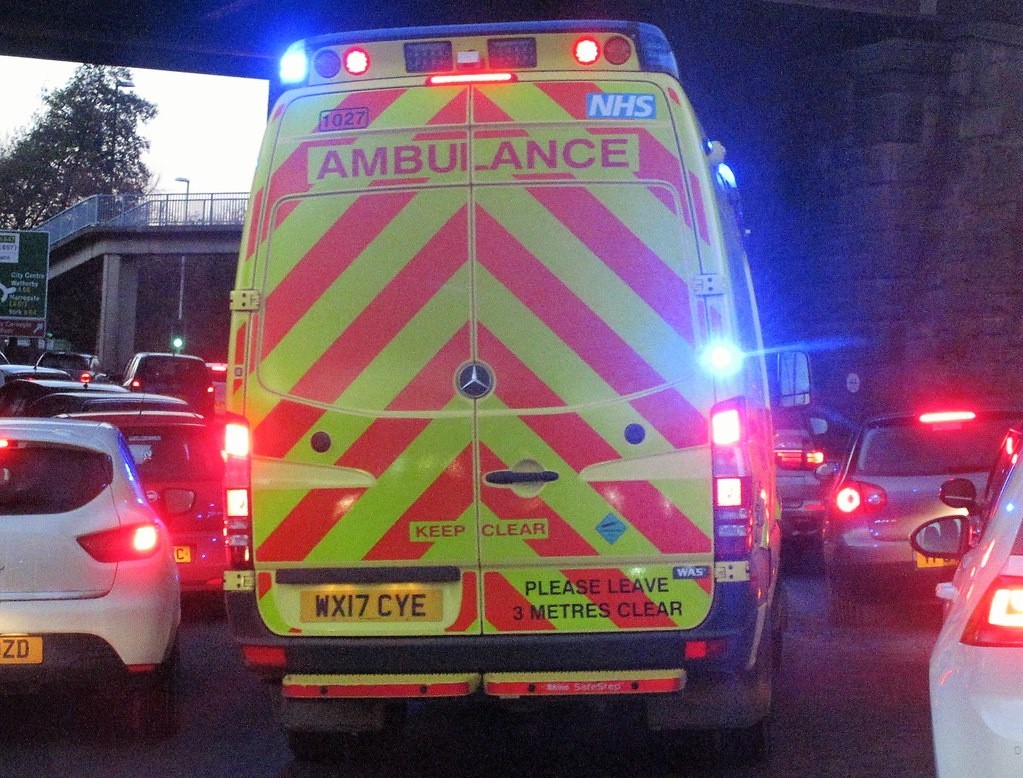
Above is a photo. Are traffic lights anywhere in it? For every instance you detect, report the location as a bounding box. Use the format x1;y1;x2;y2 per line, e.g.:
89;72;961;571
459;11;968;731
171;319;186;353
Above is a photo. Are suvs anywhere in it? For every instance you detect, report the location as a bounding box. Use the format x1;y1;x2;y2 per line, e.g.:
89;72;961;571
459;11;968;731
34;350;109;384
816;401;1022;622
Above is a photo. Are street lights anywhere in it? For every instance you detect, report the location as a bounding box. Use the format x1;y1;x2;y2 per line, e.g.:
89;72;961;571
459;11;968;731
172;178;190;351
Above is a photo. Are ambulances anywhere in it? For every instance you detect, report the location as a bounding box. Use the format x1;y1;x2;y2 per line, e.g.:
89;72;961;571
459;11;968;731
223;18;781;768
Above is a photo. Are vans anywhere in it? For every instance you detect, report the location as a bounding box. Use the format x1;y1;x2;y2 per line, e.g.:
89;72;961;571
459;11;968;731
122;353;215;421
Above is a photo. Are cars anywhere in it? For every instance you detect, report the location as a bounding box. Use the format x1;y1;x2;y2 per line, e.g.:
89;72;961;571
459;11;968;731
771;403;833;558
1;365;225;615
0;418;186;749
